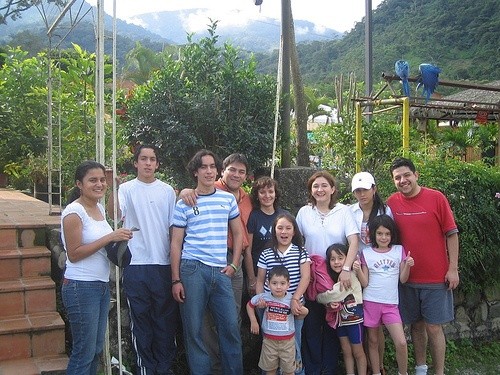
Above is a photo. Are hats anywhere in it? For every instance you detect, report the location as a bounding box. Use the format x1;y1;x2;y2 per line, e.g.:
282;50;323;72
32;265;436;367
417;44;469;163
351;172;375;192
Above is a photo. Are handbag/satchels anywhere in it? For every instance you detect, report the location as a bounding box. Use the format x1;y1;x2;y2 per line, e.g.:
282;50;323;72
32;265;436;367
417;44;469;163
106;220;139;269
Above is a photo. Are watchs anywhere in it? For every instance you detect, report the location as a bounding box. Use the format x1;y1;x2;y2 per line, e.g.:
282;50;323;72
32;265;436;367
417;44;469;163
342;266;351;272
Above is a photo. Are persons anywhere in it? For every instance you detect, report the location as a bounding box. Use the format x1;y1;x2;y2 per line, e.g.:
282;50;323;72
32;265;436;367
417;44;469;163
171;151;243;375
316;243;367;375
347;172;394;375
61;160;133;375
297;171;360;375
353;214;414;375
243;176;291;375
179;154;253;375
106;144;177;375
256;214;312;375
385;157;460;375
246;265;309;375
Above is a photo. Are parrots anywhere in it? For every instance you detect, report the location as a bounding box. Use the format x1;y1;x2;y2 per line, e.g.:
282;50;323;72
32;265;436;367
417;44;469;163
415;63;441;107
394;59;411;97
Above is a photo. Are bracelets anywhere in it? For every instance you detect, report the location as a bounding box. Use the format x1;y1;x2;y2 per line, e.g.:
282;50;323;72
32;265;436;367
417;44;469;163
248;281;256;287
171;279;181;285
290;298;298;301
231;264;238;272
